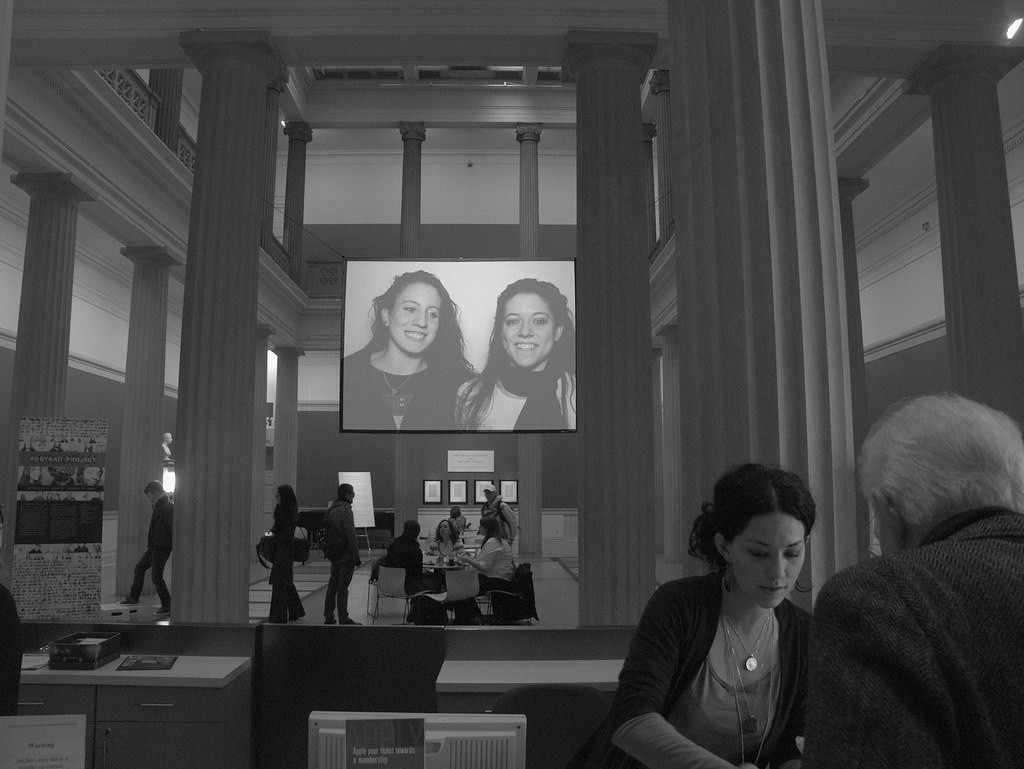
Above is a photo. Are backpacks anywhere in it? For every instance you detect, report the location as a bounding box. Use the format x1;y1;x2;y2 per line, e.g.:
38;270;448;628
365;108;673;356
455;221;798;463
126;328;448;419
317;504;347;560
368;554;389;584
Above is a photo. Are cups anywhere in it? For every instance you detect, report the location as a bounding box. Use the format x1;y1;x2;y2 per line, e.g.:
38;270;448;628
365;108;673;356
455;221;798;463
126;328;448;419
449;558;454;566
437;557;443;566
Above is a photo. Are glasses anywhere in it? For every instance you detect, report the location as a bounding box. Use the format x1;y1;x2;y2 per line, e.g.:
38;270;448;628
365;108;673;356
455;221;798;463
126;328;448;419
349;492;355;497
862;510;883;559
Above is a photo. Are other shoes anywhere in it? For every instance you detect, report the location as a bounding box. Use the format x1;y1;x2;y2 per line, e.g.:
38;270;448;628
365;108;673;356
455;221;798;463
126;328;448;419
157;607;171;616
120;598;139;605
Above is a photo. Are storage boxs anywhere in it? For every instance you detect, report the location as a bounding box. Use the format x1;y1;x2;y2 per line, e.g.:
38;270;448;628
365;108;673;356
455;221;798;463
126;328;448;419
49;631;121;669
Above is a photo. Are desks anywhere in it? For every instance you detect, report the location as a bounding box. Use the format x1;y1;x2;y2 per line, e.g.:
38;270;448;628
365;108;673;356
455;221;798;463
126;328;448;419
419;528;482;588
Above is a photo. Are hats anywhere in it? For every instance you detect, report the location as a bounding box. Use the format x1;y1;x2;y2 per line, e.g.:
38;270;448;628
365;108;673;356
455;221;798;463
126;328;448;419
484;484;496;492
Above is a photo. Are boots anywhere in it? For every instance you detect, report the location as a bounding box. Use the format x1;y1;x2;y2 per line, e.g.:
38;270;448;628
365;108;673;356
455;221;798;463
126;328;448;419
337;596;362;624
324;594;337;624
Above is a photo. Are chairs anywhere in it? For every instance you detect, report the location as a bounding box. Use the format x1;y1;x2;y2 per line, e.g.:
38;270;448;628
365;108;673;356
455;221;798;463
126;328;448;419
489;682;612;769
367;556;534;625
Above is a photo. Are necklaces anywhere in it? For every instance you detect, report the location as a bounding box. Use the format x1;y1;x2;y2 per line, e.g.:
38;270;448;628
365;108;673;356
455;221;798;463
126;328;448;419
721;598;775;768
382;346;423;395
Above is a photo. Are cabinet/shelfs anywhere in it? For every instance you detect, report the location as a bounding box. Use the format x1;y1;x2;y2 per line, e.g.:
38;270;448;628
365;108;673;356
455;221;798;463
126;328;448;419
436;659;626;769
19;652;253;769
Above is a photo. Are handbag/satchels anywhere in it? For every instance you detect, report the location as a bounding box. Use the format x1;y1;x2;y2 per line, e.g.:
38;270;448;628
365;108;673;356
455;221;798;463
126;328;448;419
258;526;309;564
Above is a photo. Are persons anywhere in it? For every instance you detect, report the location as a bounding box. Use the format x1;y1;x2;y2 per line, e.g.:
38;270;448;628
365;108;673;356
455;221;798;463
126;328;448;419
455;278;576;431
324;483;362;625
447;506;471;544
162;432;172;457
451;515;516;624
385;520;443;625
0;507;23;716
118;480;174;614
798;379;1024;769
564;461;816;769
481;485;517;568
426;519;466;569
268;485;306;624
342;269;480;430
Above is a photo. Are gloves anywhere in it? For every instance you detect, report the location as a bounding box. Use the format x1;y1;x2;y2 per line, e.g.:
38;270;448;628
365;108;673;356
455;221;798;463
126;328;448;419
356;561;361;567
